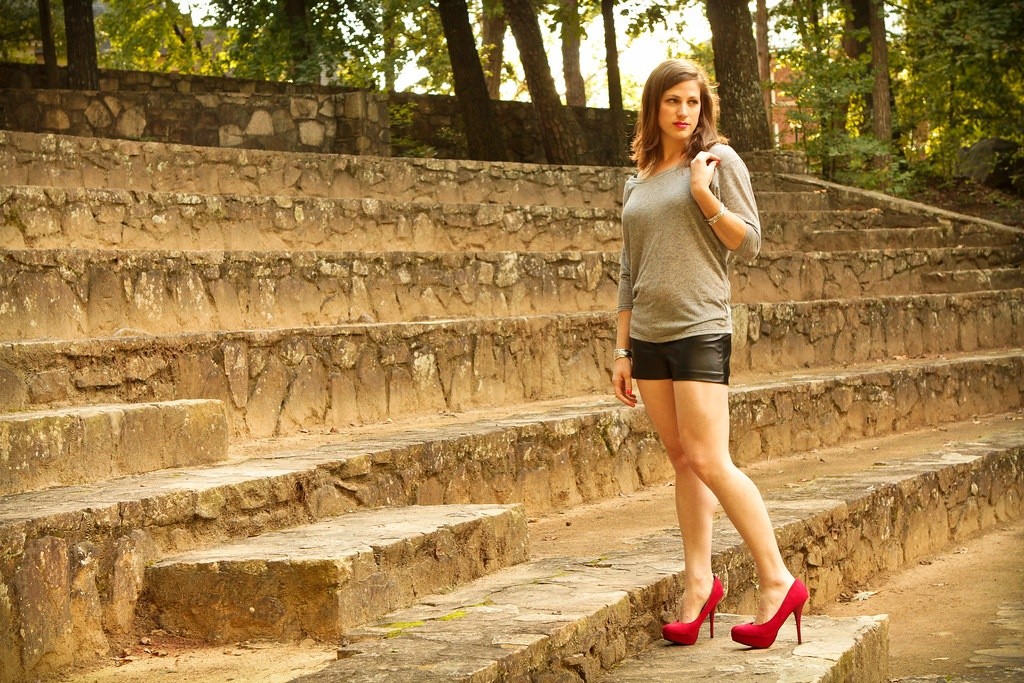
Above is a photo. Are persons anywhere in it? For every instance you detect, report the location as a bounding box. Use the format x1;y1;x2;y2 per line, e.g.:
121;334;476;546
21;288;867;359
609;59;809;648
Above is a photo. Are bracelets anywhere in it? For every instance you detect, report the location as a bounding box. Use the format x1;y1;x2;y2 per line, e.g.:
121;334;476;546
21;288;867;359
704;201;727;226
612;348;632;361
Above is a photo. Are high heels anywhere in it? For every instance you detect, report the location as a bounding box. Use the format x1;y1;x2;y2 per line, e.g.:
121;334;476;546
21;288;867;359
732;576;808;650
661;575;723;643
